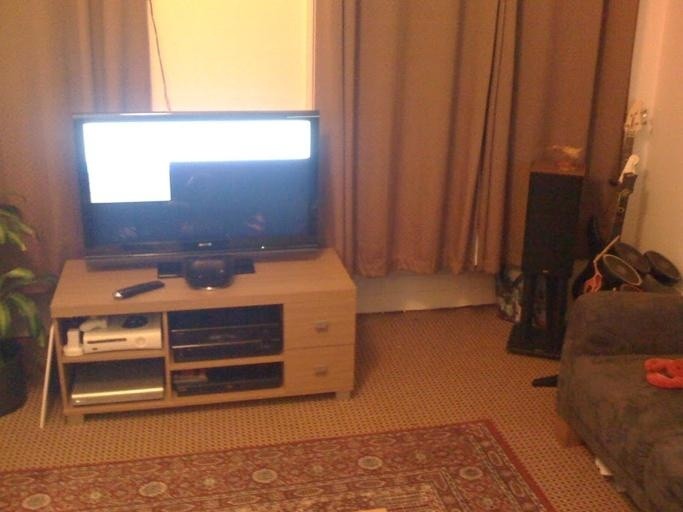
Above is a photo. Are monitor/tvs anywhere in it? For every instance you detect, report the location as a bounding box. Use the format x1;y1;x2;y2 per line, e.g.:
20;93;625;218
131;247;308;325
71;108;322;290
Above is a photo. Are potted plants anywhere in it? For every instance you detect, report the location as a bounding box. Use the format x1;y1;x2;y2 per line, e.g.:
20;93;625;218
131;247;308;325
0;188;58;418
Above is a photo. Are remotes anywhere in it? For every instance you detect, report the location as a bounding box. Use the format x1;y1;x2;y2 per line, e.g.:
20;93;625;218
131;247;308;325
112;280;165;298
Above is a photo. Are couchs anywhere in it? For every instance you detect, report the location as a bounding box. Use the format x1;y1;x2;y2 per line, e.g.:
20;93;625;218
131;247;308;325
555;291;682;511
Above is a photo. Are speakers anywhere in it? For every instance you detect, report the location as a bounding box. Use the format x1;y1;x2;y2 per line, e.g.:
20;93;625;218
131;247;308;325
504;170;601;358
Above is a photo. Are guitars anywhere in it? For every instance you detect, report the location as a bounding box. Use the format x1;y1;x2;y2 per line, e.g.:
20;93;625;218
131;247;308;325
572;96;649;300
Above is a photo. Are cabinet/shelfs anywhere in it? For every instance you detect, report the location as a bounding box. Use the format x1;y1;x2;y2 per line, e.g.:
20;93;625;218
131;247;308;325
48;247;357;426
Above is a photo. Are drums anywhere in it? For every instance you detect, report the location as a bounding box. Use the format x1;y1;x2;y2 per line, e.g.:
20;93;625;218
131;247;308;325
645;250;681;286
601;253;642;286
613;243;649;275
620;284;640;293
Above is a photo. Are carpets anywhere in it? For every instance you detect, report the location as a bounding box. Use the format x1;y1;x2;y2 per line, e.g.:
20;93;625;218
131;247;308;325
0;418;556;511
